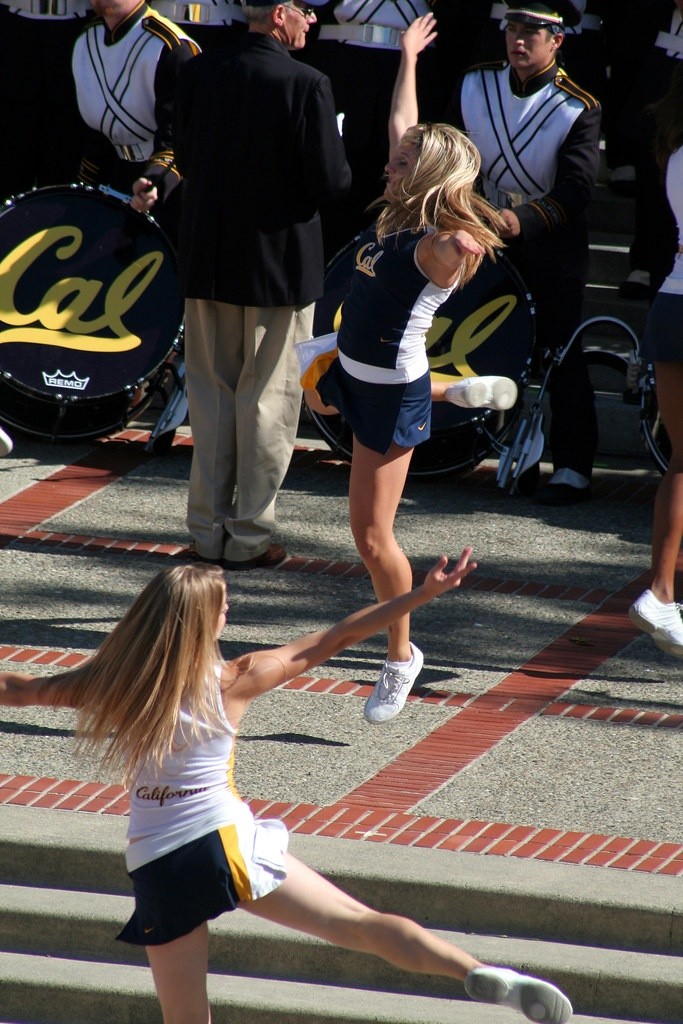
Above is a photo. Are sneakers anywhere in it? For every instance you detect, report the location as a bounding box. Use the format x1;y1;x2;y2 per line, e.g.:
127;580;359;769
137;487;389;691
363;641;424;724
628;589;683;658
446;375;518;412
463;965;574;1024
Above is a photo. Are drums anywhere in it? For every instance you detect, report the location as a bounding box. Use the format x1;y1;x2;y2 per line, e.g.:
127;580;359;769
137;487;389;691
0;178;188;446
303;216;537;479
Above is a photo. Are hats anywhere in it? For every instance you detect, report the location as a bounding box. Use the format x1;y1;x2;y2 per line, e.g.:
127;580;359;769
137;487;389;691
246;0;329;6
503;0;582;32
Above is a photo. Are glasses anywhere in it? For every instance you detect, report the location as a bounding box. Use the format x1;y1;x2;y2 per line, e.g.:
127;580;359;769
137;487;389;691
298;7;316;15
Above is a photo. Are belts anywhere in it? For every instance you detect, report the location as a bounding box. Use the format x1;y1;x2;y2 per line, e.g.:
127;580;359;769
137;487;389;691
114;135;158;161
0;0;90;16
150;4;250;23
317;23;402;48
481;180;551;211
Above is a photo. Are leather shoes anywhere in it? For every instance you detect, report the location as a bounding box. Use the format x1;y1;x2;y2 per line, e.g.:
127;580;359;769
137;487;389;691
221;543;287;569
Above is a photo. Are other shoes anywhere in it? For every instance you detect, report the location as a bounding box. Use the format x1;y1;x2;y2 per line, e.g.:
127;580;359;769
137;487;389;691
615;269;654;301
537;467;594;505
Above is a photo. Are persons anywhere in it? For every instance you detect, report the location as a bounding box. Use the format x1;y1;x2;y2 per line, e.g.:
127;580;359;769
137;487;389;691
71;0;683;660
293;13;520;725
0;547;573;1024
